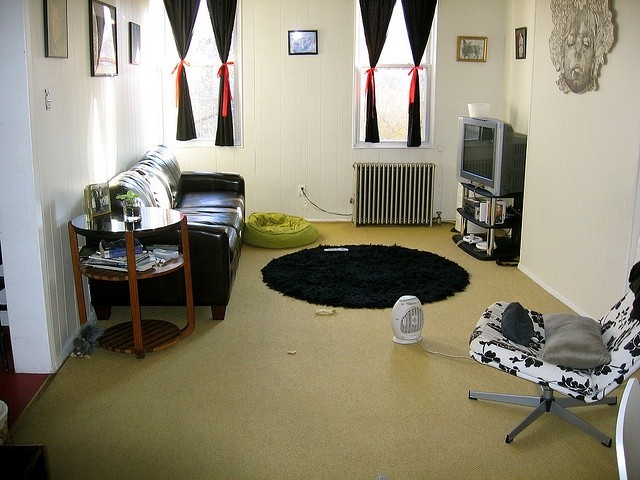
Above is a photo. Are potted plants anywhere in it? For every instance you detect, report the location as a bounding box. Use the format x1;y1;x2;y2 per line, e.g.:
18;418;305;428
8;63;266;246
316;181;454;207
116;191;143;224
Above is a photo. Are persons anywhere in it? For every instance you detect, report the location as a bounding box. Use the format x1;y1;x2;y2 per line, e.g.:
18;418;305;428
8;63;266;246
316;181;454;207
548;0;614;94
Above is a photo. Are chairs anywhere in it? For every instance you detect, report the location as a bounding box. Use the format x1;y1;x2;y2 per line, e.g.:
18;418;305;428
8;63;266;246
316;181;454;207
466;261;639;448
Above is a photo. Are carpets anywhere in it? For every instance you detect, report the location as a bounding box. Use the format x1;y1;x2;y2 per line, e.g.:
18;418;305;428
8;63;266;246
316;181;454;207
260;242;471;311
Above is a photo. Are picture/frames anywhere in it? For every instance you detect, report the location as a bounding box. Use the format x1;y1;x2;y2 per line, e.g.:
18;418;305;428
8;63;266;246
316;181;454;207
456;35;489;63
42;0;69;59
88;0;120;78
127;22;142;64
287;30;319;55
515;27;527;59
82;181;112;223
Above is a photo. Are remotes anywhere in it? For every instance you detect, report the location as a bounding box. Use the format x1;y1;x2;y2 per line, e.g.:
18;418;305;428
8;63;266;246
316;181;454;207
153;248;179;259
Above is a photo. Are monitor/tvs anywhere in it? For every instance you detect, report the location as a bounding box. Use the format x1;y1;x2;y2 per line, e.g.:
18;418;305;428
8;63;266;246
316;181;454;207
455;117;527;197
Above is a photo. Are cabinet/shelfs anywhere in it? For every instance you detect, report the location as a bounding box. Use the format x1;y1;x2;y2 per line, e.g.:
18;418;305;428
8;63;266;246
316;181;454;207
451;182;522;262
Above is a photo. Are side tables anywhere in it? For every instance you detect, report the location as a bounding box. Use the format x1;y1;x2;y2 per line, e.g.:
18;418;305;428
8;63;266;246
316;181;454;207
66;205;197;359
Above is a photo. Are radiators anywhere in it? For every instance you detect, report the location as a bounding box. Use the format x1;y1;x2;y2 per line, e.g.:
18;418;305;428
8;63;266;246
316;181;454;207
350;161;437;227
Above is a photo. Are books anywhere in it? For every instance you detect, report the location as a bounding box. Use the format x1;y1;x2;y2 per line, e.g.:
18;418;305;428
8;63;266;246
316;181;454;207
79;238;156;272
487;201;505;225
480;203;507;222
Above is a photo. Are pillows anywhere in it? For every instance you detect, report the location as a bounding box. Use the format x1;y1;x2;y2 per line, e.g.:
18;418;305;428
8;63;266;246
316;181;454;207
539;313;613;371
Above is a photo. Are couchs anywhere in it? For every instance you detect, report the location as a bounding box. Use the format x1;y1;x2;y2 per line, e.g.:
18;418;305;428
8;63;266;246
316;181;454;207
81;142;246;322
